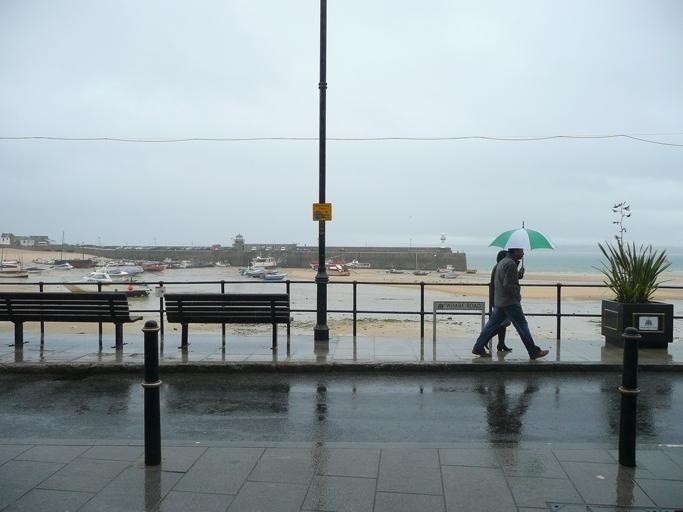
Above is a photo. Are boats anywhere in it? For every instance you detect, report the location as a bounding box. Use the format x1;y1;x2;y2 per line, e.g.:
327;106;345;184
64;262;164;297
437;265;477;278
239;257;286;280
385;269;404;274
414;270;429;275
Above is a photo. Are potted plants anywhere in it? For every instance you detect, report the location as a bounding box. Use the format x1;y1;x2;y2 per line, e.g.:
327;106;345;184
591;199;676;351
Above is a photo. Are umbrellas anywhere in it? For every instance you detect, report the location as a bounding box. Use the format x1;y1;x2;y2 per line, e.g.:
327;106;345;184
488;220;555;279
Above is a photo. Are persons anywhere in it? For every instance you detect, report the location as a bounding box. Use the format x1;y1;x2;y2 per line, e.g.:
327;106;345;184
484;250;513;352
471;248;549;360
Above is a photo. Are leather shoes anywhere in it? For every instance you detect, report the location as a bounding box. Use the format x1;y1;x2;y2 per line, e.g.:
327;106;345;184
471;345;492;357
528;350;550;360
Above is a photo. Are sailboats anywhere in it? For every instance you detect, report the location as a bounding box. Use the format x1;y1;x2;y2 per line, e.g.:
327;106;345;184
0;232;99;278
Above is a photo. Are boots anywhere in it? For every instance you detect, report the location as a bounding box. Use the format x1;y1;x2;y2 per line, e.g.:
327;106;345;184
497;327;513;351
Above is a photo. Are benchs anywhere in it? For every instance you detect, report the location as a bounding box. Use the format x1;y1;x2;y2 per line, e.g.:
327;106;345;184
0;292;143;348
162;292;293;350
